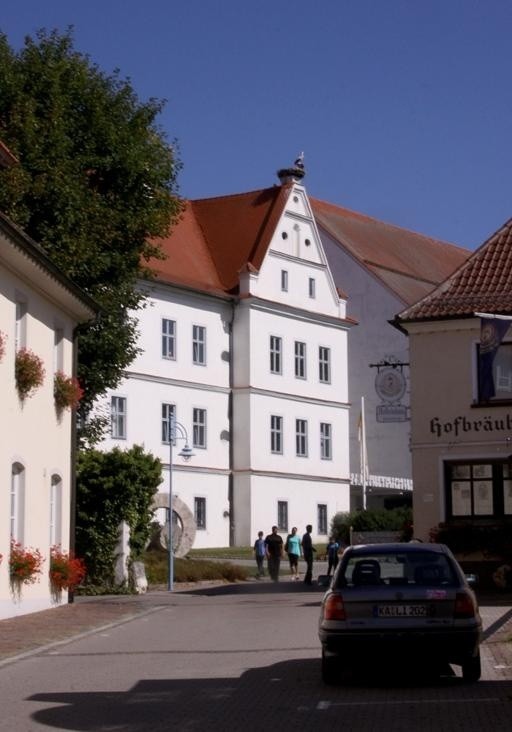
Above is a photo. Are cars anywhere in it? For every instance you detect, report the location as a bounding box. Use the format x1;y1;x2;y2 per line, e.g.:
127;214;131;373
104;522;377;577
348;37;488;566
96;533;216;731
318;522;512;684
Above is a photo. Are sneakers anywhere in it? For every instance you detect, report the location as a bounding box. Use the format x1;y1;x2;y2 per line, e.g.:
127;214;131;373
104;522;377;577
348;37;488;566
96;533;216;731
290;574;300;582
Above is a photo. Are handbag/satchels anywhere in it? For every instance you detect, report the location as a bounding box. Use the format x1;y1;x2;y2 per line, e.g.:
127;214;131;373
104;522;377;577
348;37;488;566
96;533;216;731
285;542;288;552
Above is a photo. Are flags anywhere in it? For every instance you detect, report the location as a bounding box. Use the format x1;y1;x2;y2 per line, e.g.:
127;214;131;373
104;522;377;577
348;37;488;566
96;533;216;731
476;316;511;406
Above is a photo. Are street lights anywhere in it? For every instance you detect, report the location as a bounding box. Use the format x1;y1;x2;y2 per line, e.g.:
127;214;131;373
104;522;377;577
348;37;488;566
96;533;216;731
168;411;197;589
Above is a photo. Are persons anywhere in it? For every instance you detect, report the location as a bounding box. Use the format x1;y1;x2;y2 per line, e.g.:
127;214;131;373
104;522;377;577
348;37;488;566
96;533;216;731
286;526;301;580
252;530;265;578
325;536;339;578
301;524;317;585
264;525;283;583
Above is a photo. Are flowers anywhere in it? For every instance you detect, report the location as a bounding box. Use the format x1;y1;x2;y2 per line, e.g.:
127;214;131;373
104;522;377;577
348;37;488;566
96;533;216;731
49;544;87;593
16;348;46;400
0;331;9;363
8;533;46;586
54;370;85;412
0;554;3;564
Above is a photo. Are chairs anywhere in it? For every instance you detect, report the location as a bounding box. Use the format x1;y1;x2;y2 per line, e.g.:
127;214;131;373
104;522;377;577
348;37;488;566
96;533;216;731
352;560;448;588
